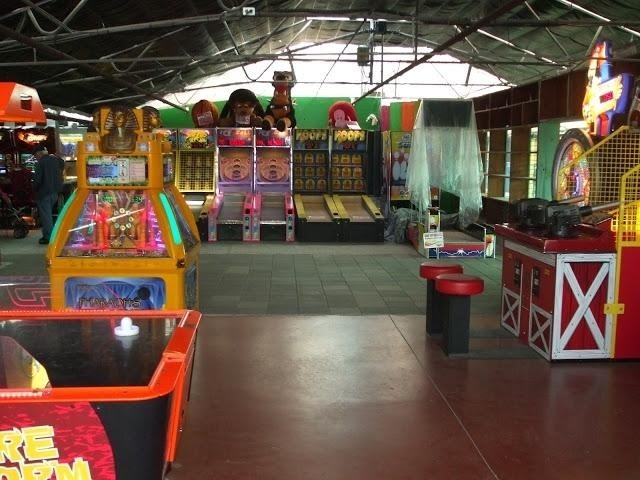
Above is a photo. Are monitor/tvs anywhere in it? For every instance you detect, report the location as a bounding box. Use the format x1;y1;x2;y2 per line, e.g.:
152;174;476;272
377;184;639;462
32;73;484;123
21;153;40;173
0;153;16;174
60;134;82;162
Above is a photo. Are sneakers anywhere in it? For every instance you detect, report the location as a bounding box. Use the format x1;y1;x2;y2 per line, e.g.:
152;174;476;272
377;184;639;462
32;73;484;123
39;236;49;244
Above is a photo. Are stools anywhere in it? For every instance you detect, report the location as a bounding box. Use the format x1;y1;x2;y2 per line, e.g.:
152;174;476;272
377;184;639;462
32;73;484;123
420;261;464;337
433;273;485;355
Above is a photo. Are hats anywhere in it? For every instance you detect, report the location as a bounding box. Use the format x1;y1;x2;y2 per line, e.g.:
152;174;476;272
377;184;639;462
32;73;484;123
32;146;45;155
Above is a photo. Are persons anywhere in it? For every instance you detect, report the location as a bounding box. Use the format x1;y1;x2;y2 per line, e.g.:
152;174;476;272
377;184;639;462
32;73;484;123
29;147;65;244
